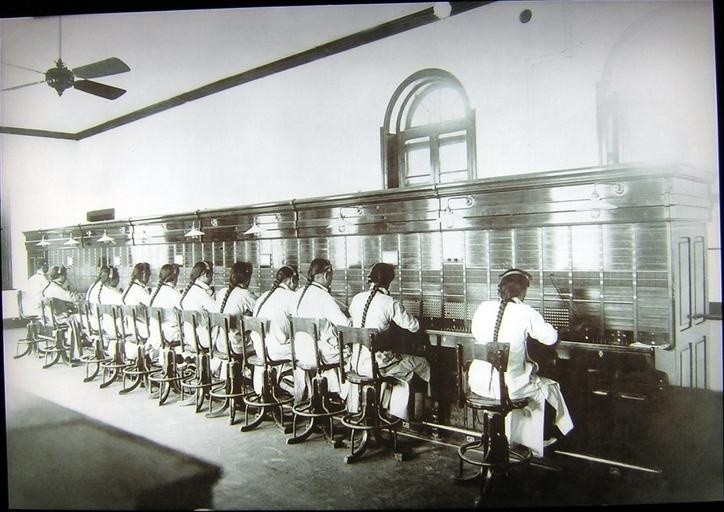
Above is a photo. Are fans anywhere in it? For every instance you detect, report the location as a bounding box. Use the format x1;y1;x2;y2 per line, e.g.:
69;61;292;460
0;16;130;104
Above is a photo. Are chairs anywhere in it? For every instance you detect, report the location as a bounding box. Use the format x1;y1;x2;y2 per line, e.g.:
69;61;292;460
455;343;535;495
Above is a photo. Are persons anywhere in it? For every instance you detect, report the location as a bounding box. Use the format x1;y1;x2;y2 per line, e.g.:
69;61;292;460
209;262;257;381
174;261;216;374
85;266;112;331
95;268;121;340
21;260;48;328
467;269;573;458
120;263;150;362
41;266;85;359
249;265;298;396
339;263;431;422
148;264;182;355
291;258;351;401
